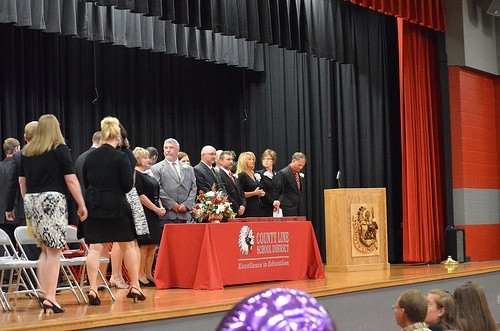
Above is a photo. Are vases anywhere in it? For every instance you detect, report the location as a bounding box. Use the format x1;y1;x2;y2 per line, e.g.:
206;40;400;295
209;214;221;223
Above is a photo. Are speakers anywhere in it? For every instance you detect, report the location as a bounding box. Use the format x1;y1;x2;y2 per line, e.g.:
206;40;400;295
446;229;465;263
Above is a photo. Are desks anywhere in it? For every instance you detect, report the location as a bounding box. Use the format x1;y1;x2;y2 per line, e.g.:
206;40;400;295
154;221;325;291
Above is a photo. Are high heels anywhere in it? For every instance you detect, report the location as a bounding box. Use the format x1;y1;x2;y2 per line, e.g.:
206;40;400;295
129;287;147;304
87;289;102;306
109;276;130;289
42;298;65;314
38;291;46;308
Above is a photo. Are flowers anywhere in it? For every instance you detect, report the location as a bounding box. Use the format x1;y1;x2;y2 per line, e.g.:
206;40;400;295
191;183;237;222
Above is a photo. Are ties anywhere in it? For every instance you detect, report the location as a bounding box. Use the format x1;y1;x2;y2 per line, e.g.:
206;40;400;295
228;170;236;184
294;172;301;193
171;162;181;183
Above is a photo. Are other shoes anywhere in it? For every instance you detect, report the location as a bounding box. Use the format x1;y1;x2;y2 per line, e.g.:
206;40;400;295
138;277;155;287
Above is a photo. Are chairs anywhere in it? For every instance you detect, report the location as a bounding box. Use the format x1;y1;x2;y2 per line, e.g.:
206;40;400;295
0;225;117;313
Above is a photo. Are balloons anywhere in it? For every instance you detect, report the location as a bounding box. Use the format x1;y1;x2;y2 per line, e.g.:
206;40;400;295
217;288;336;331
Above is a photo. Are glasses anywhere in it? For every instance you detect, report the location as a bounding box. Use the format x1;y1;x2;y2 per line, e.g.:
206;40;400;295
391;304;401;312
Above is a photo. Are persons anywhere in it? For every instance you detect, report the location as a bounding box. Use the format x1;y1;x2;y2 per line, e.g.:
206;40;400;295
76;116;152;304
392;289;433;331
0;130;308;289
5;120;39;298
454;281;498;331
424;289;462;331
18;114;88;314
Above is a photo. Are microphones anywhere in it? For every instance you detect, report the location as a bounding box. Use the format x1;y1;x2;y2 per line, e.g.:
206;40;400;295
337;170;341;179
